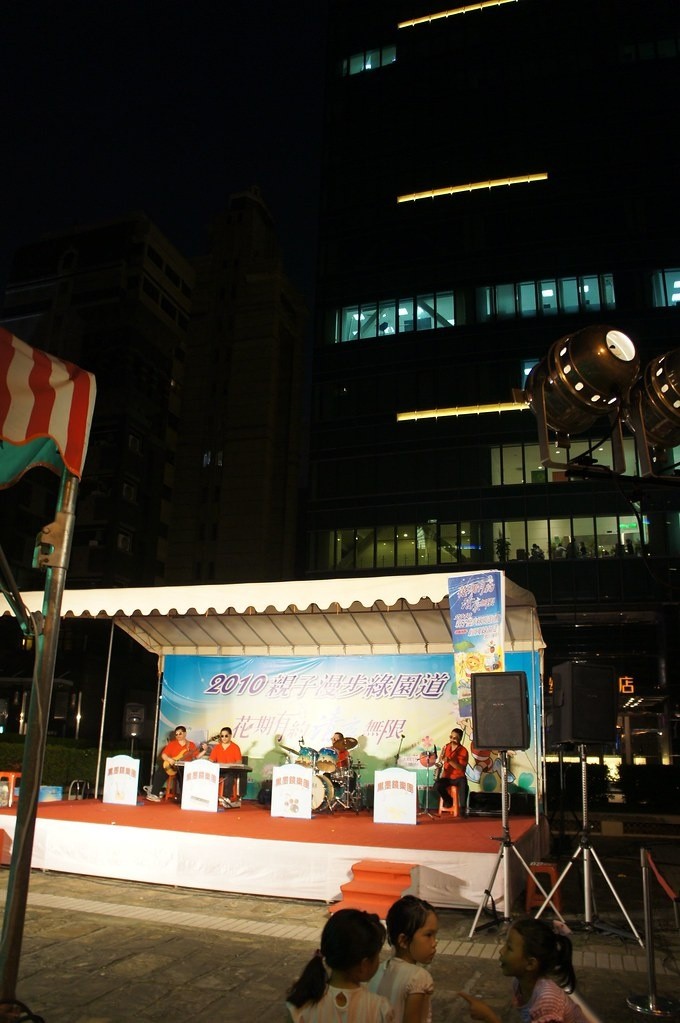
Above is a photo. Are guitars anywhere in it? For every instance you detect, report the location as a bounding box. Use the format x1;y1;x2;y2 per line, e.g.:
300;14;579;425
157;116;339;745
162;734;220;776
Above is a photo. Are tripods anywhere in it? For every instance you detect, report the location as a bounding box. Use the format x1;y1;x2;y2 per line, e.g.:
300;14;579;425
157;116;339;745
467;750;565;939
531;742;646;952
417;753;441;819
332;741;370;815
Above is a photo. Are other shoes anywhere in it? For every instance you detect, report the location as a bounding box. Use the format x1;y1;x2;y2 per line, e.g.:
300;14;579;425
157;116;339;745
146;794;161;802
224;797;230;803
460;811;468;818
444;801;453;807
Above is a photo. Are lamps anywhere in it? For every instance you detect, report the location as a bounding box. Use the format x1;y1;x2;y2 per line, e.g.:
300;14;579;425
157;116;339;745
511;323;679;482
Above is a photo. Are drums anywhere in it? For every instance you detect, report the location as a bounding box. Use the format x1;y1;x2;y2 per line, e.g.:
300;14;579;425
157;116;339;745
316;747;339;772
332;767;348;783
312;774;344;813
295;747;319;768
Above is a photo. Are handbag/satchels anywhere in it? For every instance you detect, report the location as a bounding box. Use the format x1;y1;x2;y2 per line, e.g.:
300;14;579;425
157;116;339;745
143;786;163;798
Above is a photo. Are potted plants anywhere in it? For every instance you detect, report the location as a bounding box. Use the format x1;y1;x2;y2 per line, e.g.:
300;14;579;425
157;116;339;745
494;538;512;562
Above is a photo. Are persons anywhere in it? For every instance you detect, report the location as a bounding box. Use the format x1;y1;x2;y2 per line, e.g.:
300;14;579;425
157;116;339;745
432;728;469;819
457;917;587;1023
208;727;242;804
365;895;439;1023
529;538;635;560
286;908;393;1022
145;726;208;802
322;732;357;808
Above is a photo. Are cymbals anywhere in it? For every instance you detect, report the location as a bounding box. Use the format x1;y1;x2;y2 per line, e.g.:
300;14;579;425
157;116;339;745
278;744;299;755
333;737;358;750
351;763;366;769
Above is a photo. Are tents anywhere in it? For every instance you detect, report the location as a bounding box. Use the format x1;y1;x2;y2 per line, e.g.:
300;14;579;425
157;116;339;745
1;569;549;827
1;328;95;1023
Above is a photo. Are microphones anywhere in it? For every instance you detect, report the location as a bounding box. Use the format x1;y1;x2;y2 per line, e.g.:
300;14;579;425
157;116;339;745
301;736;304;745
434;745;437;759
401;735;405;738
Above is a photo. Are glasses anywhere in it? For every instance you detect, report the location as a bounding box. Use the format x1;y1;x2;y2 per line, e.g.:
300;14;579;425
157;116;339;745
450;735;459;740
220;735;230;737
175;732;184;736
331;738;336;741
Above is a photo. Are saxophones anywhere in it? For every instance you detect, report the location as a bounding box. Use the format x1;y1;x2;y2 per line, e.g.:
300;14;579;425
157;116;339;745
432;741;453;782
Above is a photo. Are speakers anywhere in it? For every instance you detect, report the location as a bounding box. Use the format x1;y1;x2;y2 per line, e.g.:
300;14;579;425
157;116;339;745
470;672;530;751
551;662;618;752
124;703;146;739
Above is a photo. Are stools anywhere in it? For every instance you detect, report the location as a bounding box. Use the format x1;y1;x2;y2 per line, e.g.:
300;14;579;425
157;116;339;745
0;771;22;807
218;777;238;809
165;774;180;801
439;786;462;817
526;861;561;915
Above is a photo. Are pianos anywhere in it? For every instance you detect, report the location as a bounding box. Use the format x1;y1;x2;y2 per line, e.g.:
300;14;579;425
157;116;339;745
174;760;253;810
